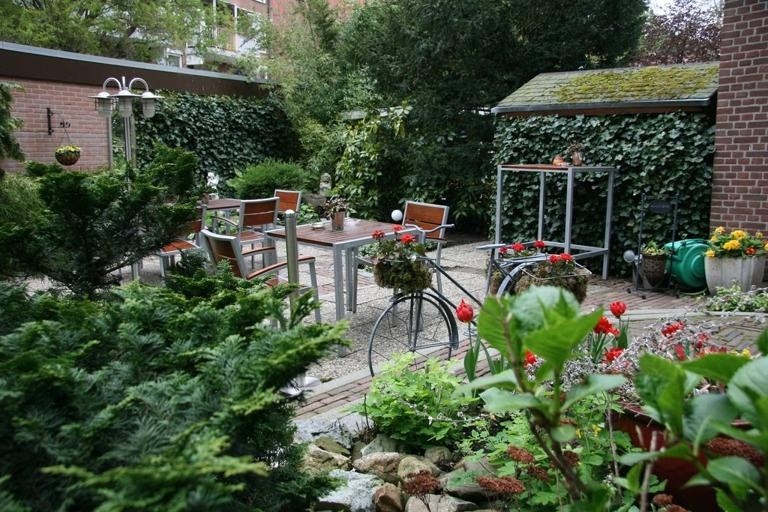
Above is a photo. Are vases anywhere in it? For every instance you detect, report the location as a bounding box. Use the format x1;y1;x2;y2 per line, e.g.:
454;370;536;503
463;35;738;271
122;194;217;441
705;256;765;296
644;255;665;276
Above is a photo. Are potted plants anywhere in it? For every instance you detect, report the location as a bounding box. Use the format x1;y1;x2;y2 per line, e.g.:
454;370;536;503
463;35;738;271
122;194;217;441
323;194;353;230
568;139;585;165
53;144;81;166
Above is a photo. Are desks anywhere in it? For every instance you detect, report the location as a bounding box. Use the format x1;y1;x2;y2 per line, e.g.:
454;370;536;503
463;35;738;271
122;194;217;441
495;163;616;284
264;212;416;356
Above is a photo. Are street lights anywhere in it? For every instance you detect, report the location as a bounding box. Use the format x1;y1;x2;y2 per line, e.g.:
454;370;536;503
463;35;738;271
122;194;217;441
88;72;163;283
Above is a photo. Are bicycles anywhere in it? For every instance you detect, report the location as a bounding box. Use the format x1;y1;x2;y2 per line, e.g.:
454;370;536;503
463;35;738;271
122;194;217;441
363;219;592;378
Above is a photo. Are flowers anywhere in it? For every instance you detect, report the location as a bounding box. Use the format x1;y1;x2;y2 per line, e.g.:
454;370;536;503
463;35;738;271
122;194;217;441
370;225;432;296
702;226;768;261
487;239;587;305
641;240;665;256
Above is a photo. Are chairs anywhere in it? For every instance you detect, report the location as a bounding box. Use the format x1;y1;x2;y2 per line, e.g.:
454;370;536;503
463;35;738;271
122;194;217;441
154;187;320;347
356;198;452;322
